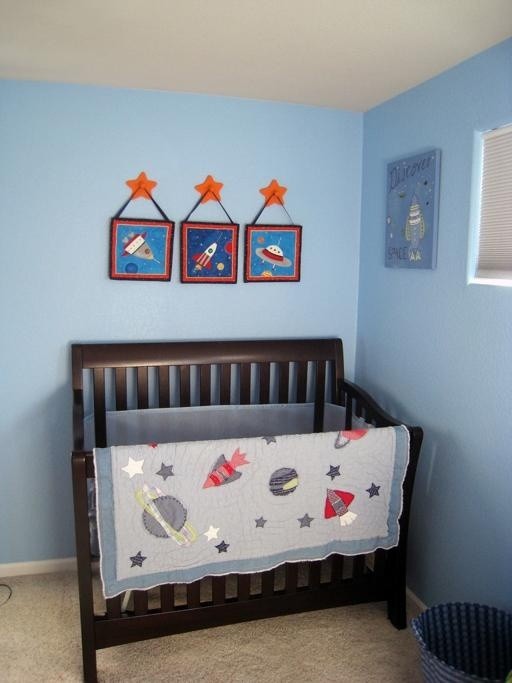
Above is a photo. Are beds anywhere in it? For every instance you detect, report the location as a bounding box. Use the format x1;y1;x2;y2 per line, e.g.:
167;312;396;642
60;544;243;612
71;339;423;683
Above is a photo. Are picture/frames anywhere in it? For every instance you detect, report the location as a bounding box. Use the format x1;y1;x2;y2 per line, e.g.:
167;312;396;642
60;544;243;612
109;217;175;281
181;222;239;283
384;148;442;270
244;225;302;282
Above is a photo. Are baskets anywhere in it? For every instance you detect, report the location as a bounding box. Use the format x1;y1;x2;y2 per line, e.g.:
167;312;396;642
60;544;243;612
411;602;512;682
